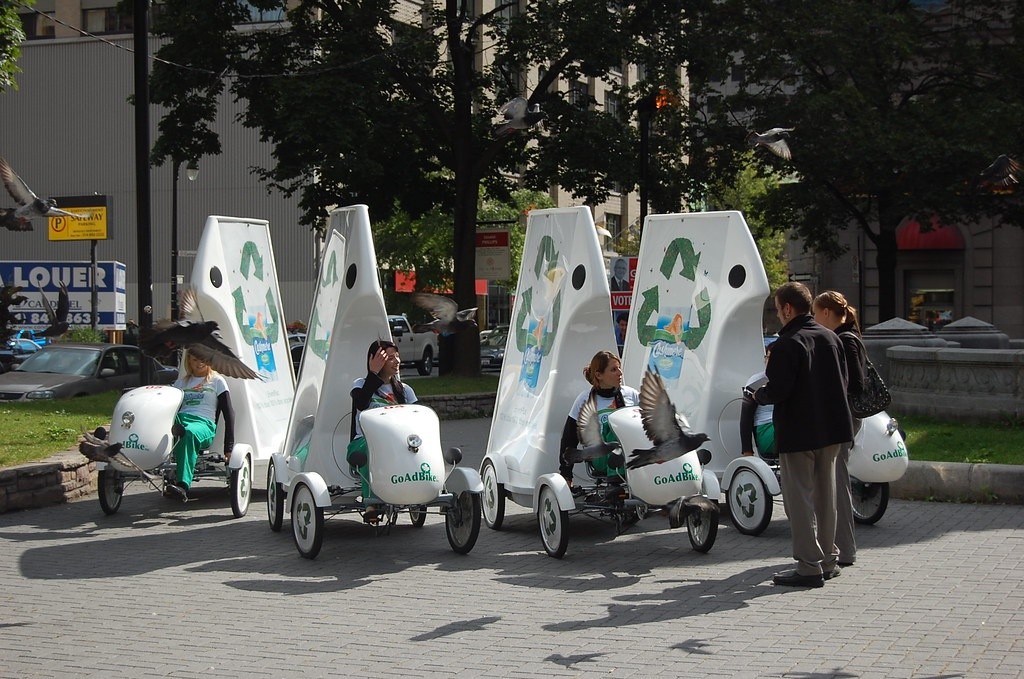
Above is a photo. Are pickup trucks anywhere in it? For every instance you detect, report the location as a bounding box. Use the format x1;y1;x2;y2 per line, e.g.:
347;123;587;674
385;315;442;375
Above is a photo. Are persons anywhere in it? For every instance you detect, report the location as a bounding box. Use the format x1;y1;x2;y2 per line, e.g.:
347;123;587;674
616;312;629;358
752;282;854;587
167;348;235;502
559;351;642;489
740;341;779;459
812;290;866;566
347;340;419;518
608;259;629;291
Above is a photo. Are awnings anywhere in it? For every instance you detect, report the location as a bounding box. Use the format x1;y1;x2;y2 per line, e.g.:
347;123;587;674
895;209;965;250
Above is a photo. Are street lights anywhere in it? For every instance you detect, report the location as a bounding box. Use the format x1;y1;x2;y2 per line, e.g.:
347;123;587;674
171;143;200;322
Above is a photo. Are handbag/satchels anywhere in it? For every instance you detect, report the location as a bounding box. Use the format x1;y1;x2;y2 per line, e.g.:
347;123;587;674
838;332;891;418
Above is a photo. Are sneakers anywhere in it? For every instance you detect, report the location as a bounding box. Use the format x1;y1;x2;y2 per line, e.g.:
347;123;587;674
823;571;840;580
774;571;824;588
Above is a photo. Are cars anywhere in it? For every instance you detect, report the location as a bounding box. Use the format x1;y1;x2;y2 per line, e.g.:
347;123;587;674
0;338;181;402
480;325;510;370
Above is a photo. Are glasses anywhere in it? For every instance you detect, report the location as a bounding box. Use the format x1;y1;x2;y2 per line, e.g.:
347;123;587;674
764;355;770;359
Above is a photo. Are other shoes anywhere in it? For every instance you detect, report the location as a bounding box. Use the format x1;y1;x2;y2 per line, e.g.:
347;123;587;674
94;427;106;439
605;485;627;500
363;511;377;522
167;483;188;504
